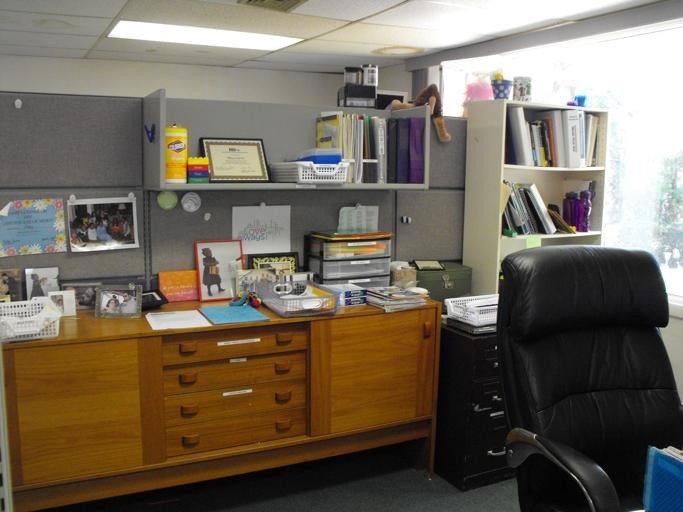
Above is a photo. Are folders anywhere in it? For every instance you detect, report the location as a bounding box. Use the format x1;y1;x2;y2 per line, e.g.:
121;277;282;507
388;118;422;183
511;181;559;235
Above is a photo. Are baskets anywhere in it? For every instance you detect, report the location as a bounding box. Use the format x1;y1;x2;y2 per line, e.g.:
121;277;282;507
444;294;500;327
268;159;350;185
0;298;61;343
254;279;342;318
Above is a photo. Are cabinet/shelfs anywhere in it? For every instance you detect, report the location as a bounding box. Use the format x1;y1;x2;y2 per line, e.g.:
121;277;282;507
433;319;517;492
302;231;393;287
461;99;609;296
0;282;442;511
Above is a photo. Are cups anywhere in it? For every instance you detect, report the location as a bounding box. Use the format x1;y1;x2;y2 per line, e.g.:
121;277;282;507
343;66;364;87
575;95;586;106
490;80;512;100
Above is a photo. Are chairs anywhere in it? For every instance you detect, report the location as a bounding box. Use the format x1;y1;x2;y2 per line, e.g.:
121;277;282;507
496;244;682;511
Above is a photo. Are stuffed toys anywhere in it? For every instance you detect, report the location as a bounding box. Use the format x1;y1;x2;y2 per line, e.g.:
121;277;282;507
385;83;451;142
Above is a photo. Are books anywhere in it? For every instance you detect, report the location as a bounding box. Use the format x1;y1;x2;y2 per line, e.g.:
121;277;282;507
316;107;429;184
643;444;683;512
364;284;429;314
503;104;600;236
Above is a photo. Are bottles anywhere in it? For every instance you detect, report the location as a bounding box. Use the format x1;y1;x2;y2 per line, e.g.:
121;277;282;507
361;63;379;87
562;190;578;226
573;190;592;232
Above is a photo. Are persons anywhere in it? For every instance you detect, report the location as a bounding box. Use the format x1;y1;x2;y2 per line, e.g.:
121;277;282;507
70;207;134;248
2;274;18;302
102;292;138;314
201;248;226;296
29;274;47;299
